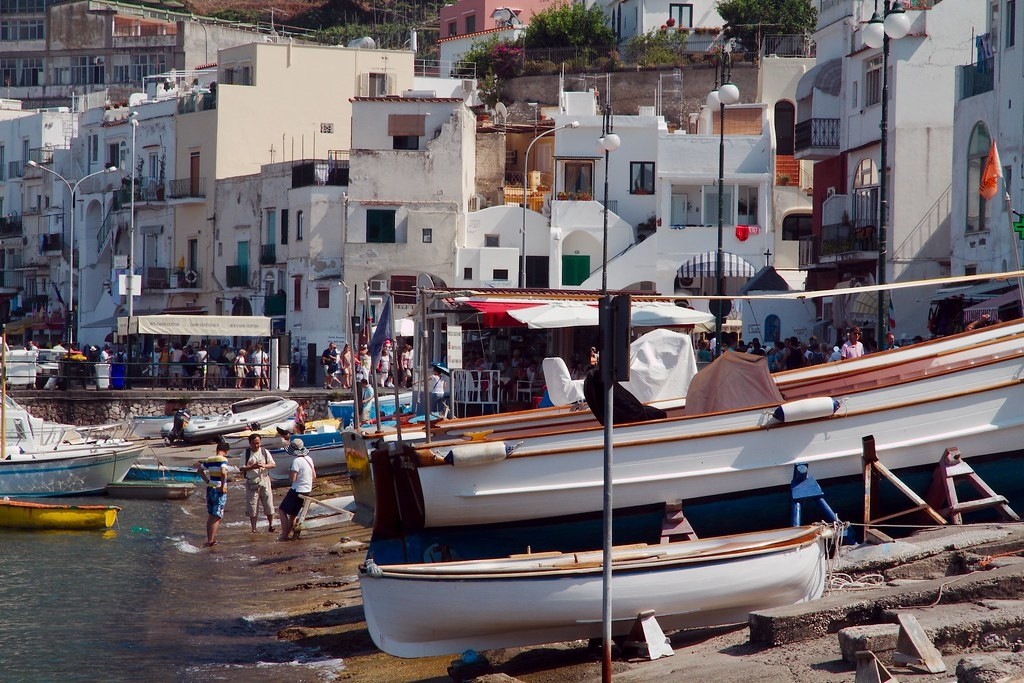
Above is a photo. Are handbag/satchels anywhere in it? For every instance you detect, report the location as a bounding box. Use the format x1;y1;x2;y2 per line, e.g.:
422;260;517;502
248;477;263;489
328;362;338;374
405;369;412;377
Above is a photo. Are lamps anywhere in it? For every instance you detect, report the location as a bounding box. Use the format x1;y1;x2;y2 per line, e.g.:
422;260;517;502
216;295;267;304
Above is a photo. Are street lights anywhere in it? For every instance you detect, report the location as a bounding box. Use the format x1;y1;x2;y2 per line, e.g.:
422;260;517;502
338;279;348;349
521;121;579;288
27;161;117;350
708;44;740;358
863;0;911;352
129;118;139;313
596;102;619;683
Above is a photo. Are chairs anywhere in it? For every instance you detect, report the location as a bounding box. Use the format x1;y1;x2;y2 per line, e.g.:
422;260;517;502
474;371;544;409
849;225;875;251
140;180;158;199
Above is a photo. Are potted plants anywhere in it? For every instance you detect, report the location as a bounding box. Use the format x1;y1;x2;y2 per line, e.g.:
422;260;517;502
537;182;551;190
635;186;649;195
695;27;721;34
678;25;690;35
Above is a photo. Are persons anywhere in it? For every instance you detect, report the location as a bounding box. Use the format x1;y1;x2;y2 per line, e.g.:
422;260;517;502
196;441;229;546
361;376;375;428
21;339;270;390
293;346;302;383
412;367;448;413
318;338;417;389
276;436;316;541
238;434;278;532
441;340;596;407
964;313;1002;332
693;321;926;373
293;397;310;434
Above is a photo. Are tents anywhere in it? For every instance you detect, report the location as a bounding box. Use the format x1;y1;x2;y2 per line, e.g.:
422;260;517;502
114;309;275;391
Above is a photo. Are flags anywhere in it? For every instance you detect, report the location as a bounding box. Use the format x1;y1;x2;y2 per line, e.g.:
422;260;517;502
977;137;1004;201
367;296;394;370
356;300;372;358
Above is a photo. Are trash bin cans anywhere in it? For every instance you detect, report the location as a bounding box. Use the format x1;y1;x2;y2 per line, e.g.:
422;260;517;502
94;364;111;390
111;363;125;390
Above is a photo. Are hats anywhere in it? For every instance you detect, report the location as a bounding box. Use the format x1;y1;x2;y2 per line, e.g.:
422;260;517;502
513;349;520;354
90;346;97;351
284;438;309;457
765;345;774;353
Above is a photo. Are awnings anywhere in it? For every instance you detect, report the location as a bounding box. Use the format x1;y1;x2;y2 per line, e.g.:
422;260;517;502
675;249;755;279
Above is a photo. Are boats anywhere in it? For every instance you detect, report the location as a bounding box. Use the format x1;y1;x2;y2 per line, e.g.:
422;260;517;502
222;317;1024;563
0;389;299;502
358;523;832;658
0;500;121;530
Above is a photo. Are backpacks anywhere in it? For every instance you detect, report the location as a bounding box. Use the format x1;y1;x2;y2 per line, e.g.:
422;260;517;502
117;353;124;362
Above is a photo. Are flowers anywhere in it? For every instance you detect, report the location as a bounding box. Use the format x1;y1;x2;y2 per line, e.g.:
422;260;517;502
708;36;723;62
558;191;592;198
661;17;675;30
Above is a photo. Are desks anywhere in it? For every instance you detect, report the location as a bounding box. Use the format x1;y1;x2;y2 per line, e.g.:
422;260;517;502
738;213;754;226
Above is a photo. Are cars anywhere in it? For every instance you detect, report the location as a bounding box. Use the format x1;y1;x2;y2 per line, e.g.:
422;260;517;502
36;349;69;379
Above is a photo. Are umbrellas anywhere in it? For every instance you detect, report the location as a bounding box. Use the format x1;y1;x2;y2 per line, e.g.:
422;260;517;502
431;362;456;379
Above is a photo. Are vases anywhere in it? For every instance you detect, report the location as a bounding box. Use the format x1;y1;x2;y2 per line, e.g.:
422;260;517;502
730;53;743;61
581;196;591;200
558;197;570;201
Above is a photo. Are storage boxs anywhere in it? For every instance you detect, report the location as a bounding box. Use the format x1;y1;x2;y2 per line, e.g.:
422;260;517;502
532;396;543;408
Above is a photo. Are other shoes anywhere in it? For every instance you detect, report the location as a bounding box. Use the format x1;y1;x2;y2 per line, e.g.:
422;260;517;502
234;385;243;389
327;386;333;389
324;383;327;389
255;386;261;390
206;541;217;547
277;535;289;542
269;527;276;532
387;382;395;388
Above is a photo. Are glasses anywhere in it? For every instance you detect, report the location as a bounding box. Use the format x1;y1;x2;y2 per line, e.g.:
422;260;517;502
853;332;862;335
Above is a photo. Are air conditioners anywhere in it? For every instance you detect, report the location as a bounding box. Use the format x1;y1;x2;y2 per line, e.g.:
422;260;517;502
679;277;701;289
371;279;387;293
